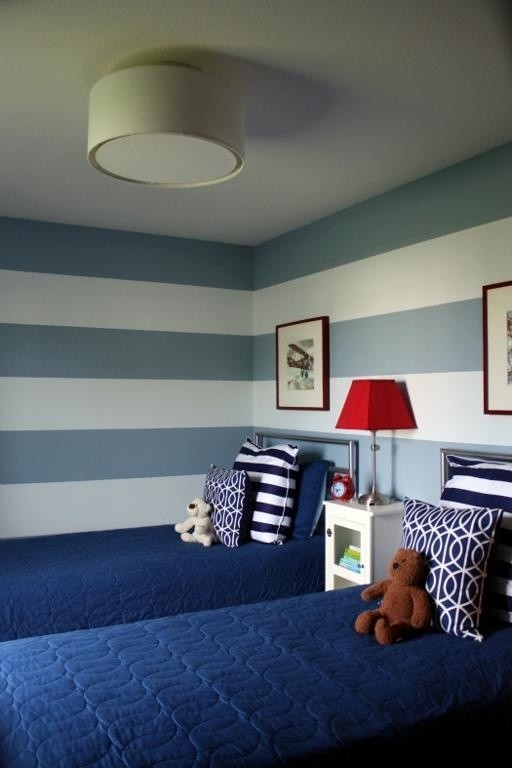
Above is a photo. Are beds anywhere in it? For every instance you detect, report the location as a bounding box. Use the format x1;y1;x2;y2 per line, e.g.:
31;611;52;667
1;448;512;768
1;433;354;642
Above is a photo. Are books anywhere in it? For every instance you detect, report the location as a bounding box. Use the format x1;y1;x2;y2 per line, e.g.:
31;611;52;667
339;545;361;573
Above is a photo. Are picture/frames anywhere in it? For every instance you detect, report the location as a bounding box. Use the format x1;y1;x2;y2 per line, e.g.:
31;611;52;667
277;316;330;411
483;281;512;415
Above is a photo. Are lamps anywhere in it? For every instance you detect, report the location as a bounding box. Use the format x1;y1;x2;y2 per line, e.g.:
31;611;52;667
336;380;418;506
87;61;246;189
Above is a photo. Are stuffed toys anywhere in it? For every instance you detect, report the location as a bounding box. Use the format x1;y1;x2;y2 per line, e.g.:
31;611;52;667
355;548;431;645
174;497;218;547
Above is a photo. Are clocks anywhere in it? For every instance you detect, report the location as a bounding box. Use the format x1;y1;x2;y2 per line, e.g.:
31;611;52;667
331;474;354;501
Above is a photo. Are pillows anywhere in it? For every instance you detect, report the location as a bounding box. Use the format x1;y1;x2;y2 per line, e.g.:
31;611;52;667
204;438;335;548
380;456;512;642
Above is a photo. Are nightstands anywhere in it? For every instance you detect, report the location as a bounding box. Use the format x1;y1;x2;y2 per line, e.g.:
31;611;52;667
322;497;405;592
